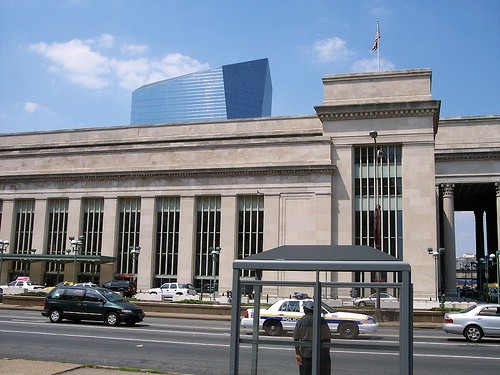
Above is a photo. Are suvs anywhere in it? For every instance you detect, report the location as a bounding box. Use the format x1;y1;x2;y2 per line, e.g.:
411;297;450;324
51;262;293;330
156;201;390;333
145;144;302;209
41;286;145;327
149;282;197;297
100;280;138;299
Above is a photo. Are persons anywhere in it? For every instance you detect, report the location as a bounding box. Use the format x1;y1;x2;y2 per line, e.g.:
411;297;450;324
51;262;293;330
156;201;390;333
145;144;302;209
438;287;446;298
292;301;332;375
462;283;468;298
128;286;133;297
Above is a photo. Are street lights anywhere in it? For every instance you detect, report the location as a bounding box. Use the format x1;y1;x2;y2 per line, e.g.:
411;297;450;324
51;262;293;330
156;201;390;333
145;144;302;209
129;246;141;276
209;246;221;291
0;239;11;279
368;128;378;249
68;236;84;285
464;250;500;304
376;149;384;250
427;247;445;301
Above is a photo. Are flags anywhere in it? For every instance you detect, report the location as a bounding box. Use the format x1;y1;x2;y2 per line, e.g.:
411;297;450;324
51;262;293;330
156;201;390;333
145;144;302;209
372;31;381;52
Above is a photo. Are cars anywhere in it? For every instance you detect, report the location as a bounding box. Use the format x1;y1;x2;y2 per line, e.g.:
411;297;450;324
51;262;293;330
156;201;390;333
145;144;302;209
442;303;500;342
353;293;399;307
0;276;46;295
240;291;379;340
200;283;219;293
42;281;97;293
457;285;500;304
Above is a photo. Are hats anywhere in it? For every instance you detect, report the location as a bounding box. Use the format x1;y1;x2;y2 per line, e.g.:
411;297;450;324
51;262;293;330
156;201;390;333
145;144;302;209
301;301;315;310
